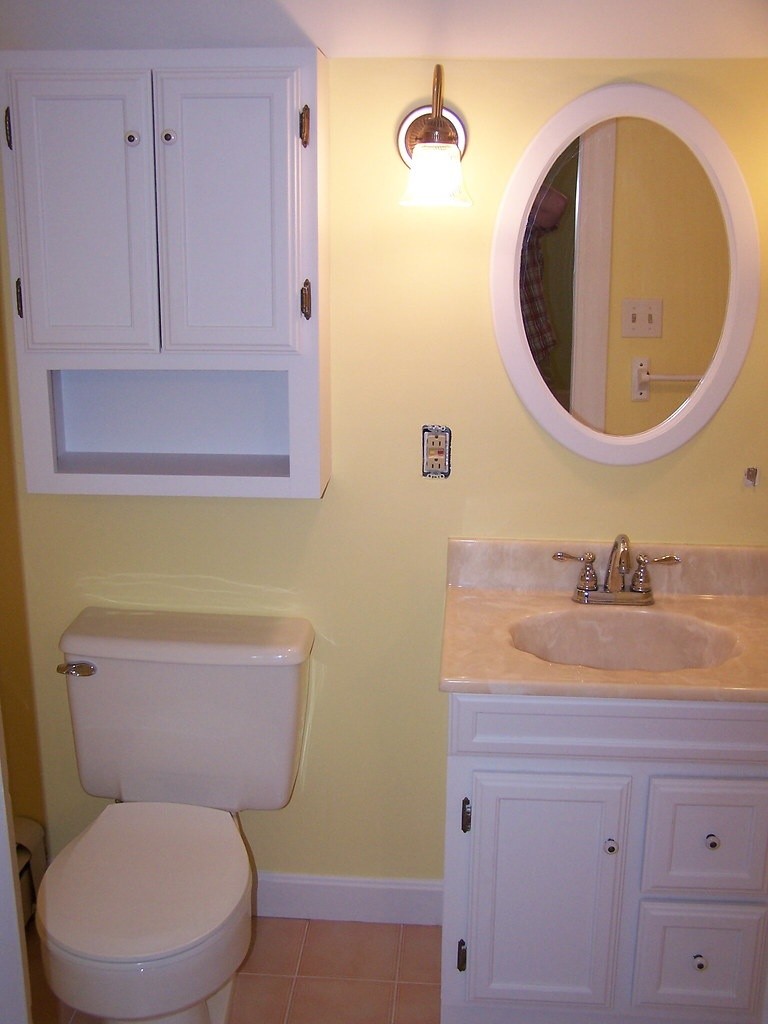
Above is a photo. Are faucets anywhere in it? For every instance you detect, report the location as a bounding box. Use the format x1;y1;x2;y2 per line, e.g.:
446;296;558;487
603;533;633;594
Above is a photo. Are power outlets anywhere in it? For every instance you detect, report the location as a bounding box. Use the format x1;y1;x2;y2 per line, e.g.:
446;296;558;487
421;424;452;479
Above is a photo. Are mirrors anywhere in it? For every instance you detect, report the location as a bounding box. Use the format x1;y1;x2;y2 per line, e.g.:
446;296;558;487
489;81;761;466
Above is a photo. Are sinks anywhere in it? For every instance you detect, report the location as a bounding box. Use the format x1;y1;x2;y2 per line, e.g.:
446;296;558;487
504;597;747;675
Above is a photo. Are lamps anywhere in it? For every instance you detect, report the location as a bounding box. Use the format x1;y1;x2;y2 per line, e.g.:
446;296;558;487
395;63;471;206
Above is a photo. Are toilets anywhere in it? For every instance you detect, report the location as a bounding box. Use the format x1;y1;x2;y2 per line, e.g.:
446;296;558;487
34;600;318;1024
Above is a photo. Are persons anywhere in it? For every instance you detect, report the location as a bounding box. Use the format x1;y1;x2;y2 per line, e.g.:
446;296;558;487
519;182;568;364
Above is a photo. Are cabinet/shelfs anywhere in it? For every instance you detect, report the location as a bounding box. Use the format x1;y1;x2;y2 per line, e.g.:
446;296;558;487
0;48;333;501
442;754;767;1024
